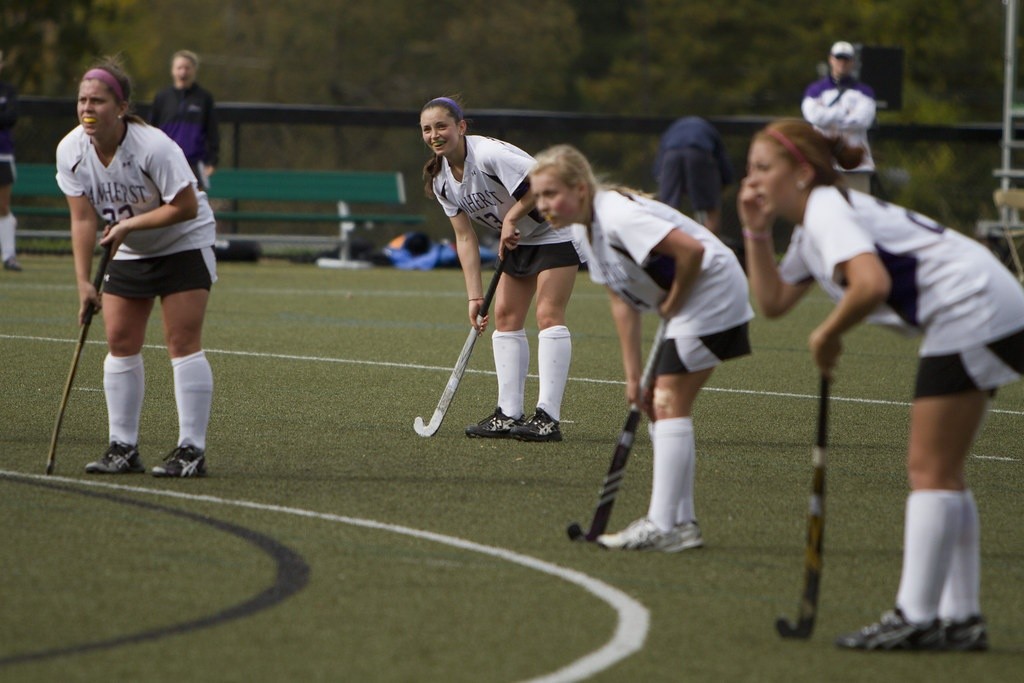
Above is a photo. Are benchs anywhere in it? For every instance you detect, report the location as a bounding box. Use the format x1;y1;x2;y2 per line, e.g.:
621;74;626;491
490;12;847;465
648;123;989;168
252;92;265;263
7;163;431;262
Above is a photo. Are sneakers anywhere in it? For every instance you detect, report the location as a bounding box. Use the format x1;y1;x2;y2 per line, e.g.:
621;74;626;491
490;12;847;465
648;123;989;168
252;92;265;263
150;444;205;477
595;519;676;551
507;409;562;442
835;608;945;653
938;614;990;653
657;521;704;552
84;441;145;473
465;407;526;439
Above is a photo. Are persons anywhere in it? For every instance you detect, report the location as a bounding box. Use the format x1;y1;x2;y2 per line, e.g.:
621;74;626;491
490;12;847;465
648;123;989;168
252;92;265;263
800;42;878;195
420;97;581;442
652;115;735;237
529;145;755;551
739;118;1024;651
149;49;220;194
0;83;25;272
56;66;217;476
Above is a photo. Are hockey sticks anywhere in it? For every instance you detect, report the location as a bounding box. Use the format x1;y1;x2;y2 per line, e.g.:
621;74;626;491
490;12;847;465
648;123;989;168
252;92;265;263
45;218;120;475
776;356;835;640
570;315;670;544
413;226;522;439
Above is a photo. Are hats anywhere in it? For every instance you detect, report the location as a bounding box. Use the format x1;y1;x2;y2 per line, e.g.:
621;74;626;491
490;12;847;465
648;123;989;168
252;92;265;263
830;41;856;57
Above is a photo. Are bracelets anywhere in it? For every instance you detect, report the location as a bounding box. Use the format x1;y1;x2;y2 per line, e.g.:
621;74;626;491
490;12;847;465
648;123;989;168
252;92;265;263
743;229;773;241
469;298;484;301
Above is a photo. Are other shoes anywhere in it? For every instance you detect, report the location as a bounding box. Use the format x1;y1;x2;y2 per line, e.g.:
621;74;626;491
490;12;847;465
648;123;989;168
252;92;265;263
5;258;23;271
